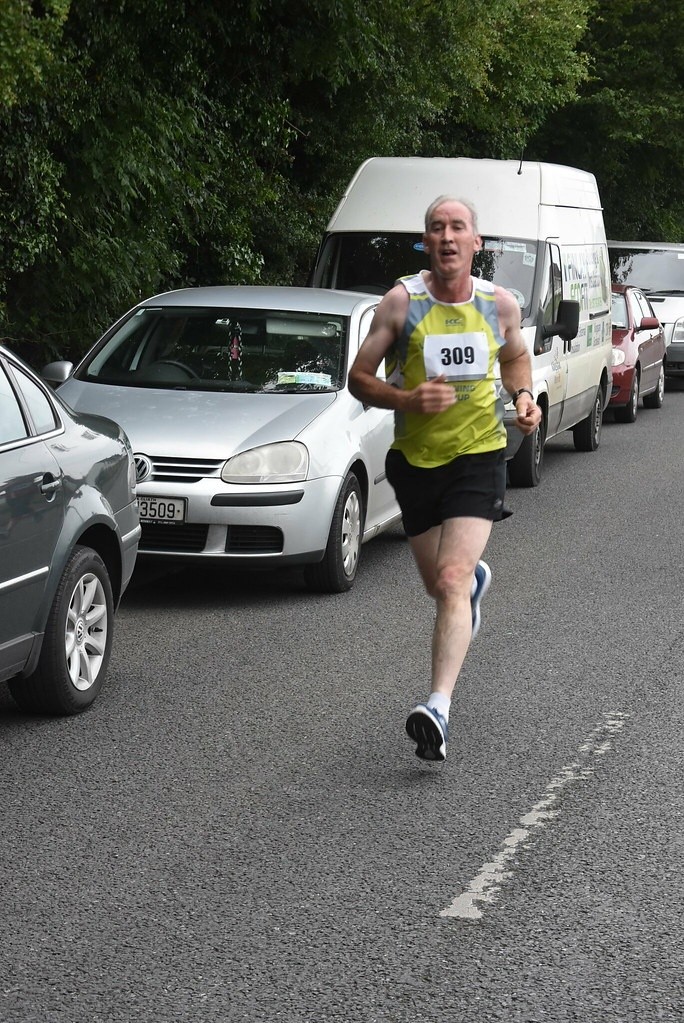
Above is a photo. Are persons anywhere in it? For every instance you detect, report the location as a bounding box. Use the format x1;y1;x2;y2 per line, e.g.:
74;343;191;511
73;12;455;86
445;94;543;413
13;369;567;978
347;193;544;759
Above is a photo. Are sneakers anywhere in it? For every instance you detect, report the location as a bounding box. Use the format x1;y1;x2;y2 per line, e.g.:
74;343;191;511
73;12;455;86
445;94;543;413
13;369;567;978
405;705;449;762
470;560;492;641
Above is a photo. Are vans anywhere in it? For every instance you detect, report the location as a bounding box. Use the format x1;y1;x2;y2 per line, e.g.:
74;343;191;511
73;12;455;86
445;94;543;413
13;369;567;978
605;239;684;378
306;153;614;485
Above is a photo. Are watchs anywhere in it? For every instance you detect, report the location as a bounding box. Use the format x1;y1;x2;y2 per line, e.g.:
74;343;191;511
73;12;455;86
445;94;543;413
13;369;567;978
510;388;534;405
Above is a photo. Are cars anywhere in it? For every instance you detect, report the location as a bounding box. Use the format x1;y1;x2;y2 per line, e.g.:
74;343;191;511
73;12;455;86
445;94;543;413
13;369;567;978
607;282;666;421
0;346;142;717
37;283;413;592
